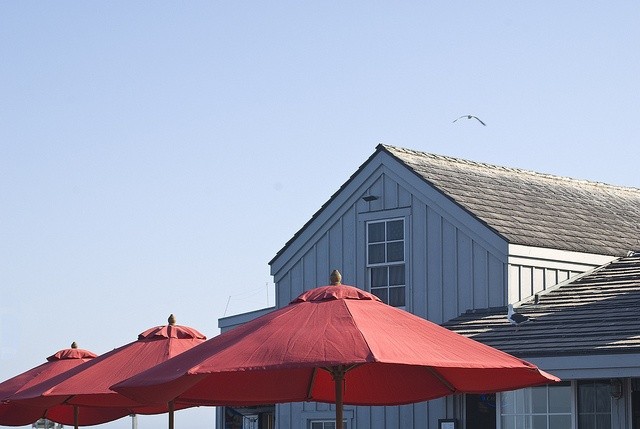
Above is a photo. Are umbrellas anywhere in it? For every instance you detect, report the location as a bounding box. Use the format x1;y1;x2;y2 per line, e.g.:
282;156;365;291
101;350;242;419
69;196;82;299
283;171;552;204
1;341;197;428
110;270;562;429
1;315;208;428
